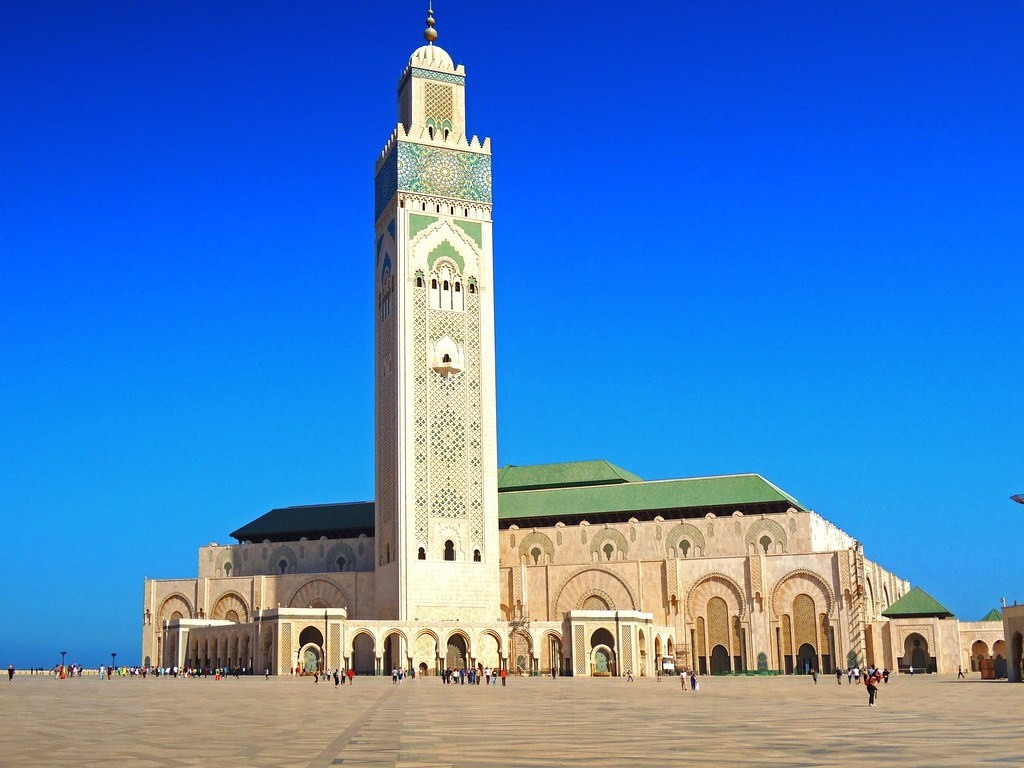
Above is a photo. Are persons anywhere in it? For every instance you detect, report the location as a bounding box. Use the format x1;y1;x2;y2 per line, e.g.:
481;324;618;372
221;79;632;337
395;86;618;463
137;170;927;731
291;666;354;689
836;667;843;685
484;667;490;685
31;664;83;679
392;667;407;685
265;668;269;680
441;666;481;685
99;663;241;681
909;666;913;677
658;670;661;682
883;668;889;683
680;669;687;691
8;665;15;681
690;671;697;690
846;665;883;686
813;669;818;686
867;671;883;706
492;669;497;684
501;668;506;686
627;670;633;682
411;668;415;679
419;669;422;679
958;665;965;679
